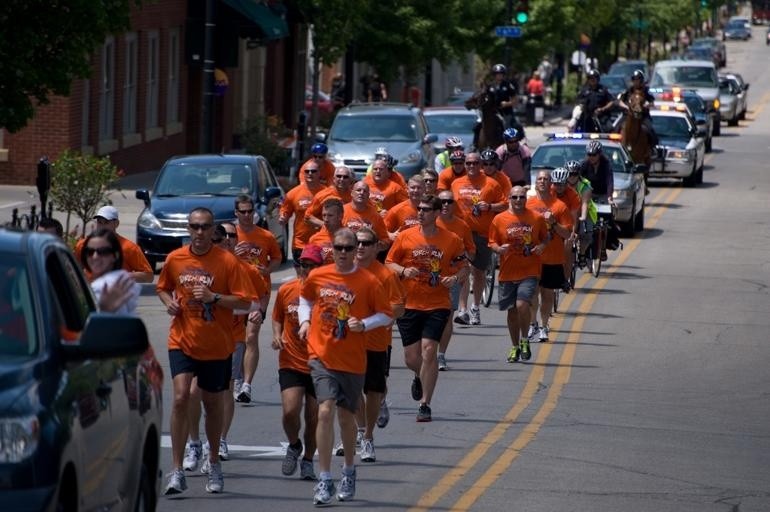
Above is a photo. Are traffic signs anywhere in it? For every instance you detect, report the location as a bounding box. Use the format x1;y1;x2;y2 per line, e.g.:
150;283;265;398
495;25;523;38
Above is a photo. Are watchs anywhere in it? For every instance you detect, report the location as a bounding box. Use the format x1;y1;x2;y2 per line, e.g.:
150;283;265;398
213;293;222;303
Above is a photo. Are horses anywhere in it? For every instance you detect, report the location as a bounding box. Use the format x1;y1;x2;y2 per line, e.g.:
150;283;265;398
464;80;509;152
574;88;606;133
622;81;653;195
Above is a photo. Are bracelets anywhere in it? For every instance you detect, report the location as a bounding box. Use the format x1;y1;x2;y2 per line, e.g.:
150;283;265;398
361;321;366;330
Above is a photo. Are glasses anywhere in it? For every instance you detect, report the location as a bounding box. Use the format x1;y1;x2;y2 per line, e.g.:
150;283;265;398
83;247;113;256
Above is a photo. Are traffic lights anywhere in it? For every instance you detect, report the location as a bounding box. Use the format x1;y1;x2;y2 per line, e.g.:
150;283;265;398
516;3;530;25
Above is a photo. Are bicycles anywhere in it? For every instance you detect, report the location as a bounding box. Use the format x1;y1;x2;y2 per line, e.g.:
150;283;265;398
547;202;624;314
477;252;496;306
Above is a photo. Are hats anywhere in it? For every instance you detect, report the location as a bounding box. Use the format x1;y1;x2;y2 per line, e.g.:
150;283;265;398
93;206;118;221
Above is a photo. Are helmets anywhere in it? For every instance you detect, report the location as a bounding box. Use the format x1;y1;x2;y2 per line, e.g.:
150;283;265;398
588;70;600;80
492;64;505;73
632;70;642;80
311;128;603;184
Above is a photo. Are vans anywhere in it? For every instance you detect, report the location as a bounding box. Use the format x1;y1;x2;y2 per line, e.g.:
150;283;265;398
135;152;292;273
326;101;440;180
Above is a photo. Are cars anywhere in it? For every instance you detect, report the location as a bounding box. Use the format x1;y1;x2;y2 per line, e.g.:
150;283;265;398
425;107;483;156
305;86;348;125
0;228;165;509
526;134;648;234
594;16;754;189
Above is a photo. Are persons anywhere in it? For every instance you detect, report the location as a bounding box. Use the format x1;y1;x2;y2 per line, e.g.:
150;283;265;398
154;206;263;498
575;69;658;194
269;243;328;484
33;217;65;240
332;70;422;108
78;228;138;318
182;146;475;476
435;131;618;363
73;205;156;284
297;225;396;503
624;24;696;78
0;264;136;349
467;54;563;145
